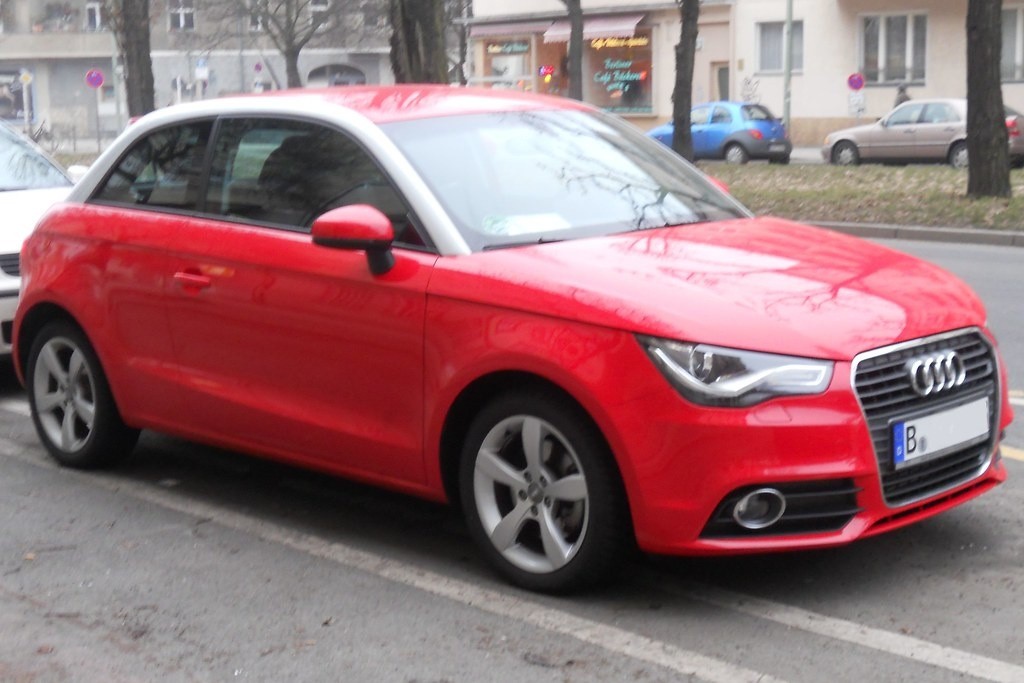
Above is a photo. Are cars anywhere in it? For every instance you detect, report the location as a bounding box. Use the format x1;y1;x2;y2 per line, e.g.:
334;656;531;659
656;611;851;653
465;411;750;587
821;97;1024;170
643;101;795;165
1;120;77;371
6;75;1018;594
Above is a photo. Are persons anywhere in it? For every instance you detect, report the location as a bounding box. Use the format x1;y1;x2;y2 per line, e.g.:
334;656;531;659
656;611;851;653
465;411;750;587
894;83;910;108
